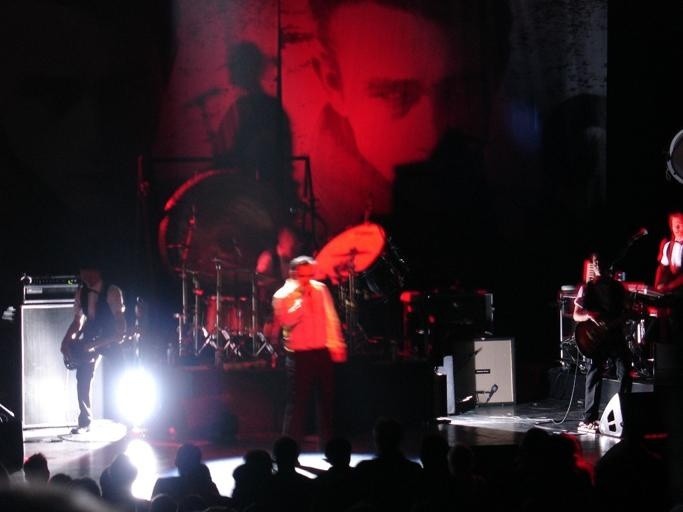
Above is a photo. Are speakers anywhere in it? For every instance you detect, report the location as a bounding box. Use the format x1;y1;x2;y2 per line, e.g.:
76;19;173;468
456;337;517;408
20;304;106;430
598;392;664;437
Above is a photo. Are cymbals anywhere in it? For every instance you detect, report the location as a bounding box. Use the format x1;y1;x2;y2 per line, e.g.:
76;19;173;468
315;221;386;286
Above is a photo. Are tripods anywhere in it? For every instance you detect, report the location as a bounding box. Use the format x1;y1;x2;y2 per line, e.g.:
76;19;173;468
163;204;279;363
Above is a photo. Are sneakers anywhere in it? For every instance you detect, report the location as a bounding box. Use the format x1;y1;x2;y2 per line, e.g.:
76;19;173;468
71;425;89;434
578;423;599;434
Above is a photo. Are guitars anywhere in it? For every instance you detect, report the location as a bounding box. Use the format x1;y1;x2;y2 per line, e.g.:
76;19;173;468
64;327;140;370
576;293;659;358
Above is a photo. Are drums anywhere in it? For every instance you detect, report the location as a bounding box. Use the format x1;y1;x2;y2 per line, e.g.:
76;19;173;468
206;292;252;335
361;234;418;302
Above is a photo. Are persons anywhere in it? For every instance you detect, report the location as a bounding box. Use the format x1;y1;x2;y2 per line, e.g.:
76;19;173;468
0;427;683;512
572;251;633;430
653;213;683;357
271;257;347;454
60;260;128;435
